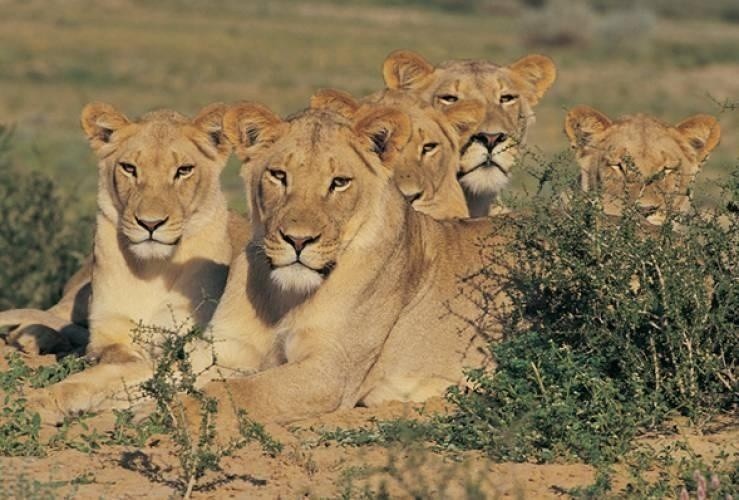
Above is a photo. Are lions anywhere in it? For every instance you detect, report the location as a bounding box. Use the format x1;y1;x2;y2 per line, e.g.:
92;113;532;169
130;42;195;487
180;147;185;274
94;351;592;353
0;46;738;429
